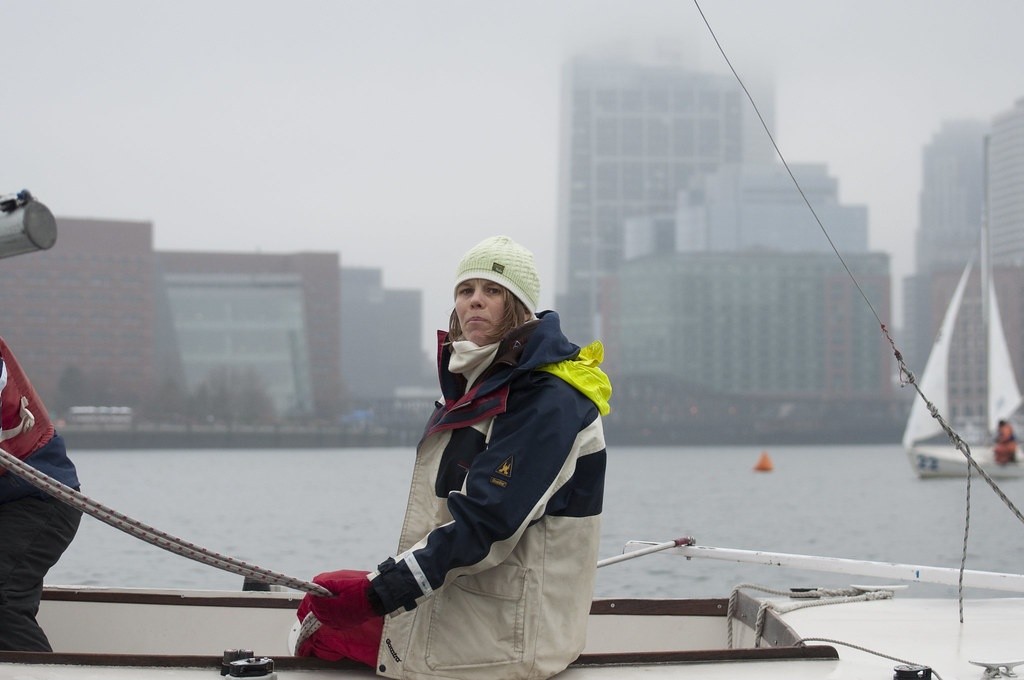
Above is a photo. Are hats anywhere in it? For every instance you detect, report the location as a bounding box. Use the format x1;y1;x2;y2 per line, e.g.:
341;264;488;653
453;236;540;320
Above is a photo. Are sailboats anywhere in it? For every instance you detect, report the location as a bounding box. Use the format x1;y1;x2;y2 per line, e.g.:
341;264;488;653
901;133;1023;479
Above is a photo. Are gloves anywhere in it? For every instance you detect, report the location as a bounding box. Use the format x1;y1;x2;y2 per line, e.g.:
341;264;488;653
305;569;380;629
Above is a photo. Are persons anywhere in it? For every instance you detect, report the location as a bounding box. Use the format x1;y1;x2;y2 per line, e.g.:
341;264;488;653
993;419;1016;463
297;232;611;680
0;335;85;653
